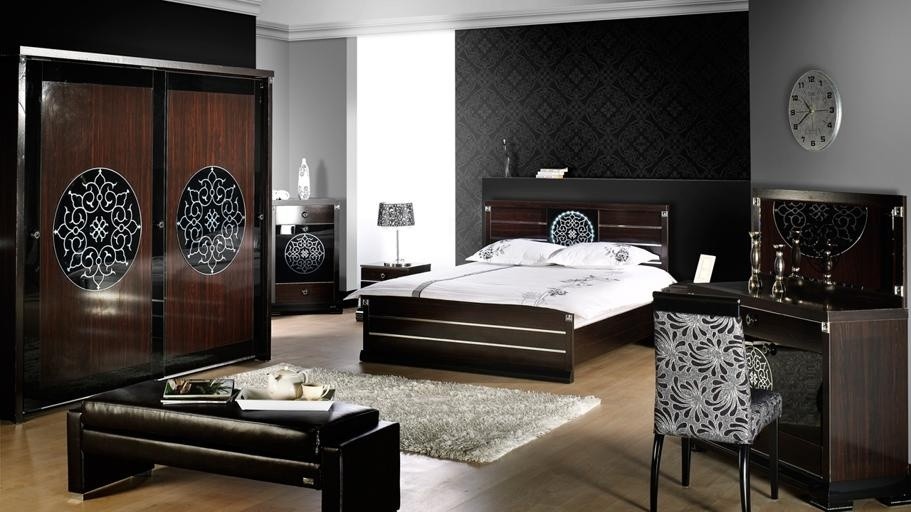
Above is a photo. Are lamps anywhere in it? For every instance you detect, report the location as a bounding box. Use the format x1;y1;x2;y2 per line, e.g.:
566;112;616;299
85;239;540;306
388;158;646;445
378;201;418;268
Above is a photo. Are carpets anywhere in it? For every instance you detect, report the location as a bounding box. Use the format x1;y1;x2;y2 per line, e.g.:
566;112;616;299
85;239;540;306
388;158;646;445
218;359;602;465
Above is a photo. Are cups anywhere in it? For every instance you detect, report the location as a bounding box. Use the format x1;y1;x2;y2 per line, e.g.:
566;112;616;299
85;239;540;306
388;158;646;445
300;382;330;401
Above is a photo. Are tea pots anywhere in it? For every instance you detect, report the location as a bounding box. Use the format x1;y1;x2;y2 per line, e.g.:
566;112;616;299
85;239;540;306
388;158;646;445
264;365;309;400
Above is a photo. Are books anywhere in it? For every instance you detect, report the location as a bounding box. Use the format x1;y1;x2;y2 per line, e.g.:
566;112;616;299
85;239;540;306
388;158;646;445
535;168;568;179
159;377;241;407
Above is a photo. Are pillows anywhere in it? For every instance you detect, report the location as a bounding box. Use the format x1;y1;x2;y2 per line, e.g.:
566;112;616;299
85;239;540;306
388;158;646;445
468;232;658;276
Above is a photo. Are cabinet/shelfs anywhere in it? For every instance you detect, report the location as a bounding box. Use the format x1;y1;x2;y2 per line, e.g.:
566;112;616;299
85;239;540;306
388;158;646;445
7;55;277;419
653;187;910;512
274;199;341;315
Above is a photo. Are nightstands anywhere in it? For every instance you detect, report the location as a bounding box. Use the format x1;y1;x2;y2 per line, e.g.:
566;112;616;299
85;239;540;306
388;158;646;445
362;263;429;317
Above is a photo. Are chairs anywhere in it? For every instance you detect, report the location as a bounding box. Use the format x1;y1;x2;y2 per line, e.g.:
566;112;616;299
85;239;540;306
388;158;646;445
644;292;788;511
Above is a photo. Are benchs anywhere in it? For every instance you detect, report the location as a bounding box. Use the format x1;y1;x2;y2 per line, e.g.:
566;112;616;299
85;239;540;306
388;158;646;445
62;380;400;512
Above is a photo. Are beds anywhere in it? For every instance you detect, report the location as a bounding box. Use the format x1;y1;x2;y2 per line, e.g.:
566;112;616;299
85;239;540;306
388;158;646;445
354;200;674;388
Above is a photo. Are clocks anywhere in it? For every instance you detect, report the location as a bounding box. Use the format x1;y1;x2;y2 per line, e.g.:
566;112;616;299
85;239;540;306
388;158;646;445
784;65;842;153
